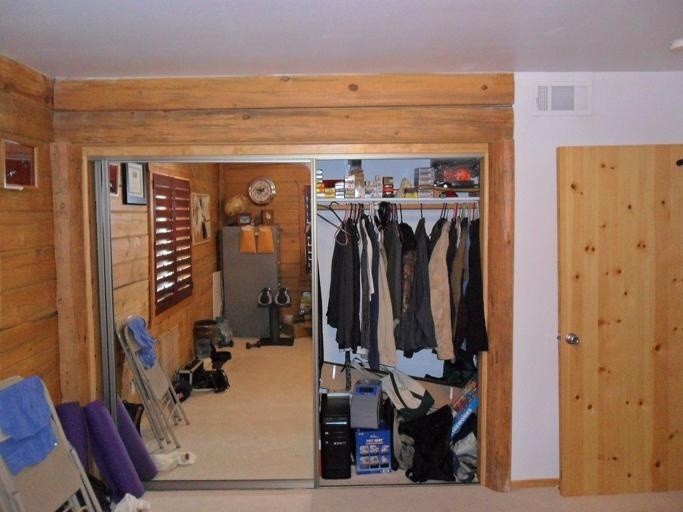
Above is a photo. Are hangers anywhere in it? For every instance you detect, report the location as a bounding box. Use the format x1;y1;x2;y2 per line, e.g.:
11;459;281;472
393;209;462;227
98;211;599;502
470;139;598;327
317;198;475;246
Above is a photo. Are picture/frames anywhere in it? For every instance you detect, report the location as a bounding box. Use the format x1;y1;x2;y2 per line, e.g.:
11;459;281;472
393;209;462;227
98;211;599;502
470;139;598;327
191;192;212;247
121;161;147;204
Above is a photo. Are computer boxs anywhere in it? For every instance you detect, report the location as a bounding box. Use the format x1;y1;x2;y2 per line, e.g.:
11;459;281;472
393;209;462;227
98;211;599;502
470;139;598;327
321;396;351;479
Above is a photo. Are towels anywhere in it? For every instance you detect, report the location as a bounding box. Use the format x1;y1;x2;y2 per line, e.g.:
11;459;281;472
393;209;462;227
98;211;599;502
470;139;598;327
0;374;59;475
128;315;157;369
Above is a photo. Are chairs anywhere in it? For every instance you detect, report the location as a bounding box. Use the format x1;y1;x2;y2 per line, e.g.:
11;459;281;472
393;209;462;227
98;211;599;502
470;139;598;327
115;313;190;451
0;374;104;512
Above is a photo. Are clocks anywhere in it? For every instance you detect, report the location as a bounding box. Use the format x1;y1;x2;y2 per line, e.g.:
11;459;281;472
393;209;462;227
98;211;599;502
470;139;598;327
248;177;275;206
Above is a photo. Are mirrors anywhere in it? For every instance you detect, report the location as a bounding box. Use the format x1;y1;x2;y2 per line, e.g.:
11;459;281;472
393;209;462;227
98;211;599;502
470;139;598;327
93;155;312;490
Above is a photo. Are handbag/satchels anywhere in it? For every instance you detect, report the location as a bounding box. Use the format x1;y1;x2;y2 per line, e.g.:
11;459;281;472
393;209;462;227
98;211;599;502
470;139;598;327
396;406;457;482
380;368;434;422
